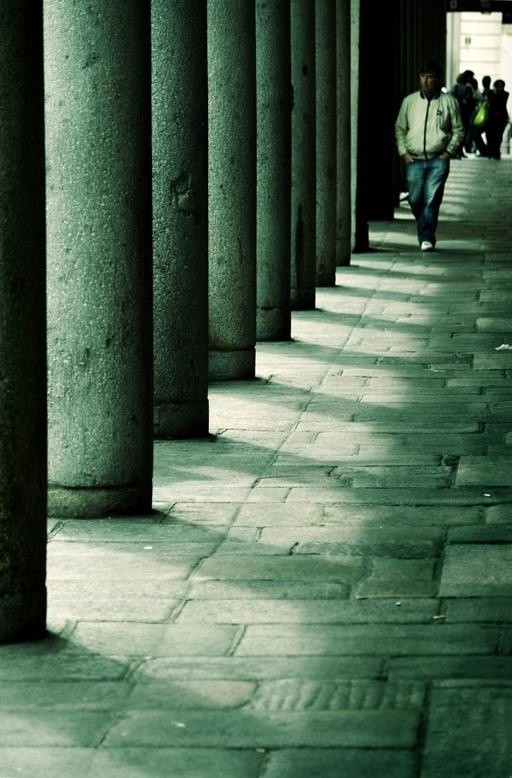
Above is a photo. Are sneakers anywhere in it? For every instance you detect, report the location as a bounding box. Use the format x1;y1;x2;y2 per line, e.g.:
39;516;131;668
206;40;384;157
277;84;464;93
420;240;433;251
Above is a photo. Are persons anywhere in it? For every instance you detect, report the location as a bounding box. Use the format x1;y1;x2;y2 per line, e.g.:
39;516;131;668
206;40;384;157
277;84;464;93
442;68;511;160
393;61;467;252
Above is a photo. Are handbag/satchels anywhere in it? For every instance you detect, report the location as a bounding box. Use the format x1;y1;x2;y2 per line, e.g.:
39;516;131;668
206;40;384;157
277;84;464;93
473;102;488;126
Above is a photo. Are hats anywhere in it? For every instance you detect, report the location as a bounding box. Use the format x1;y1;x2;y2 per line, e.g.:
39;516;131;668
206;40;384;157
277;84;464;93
420;62;441;79
